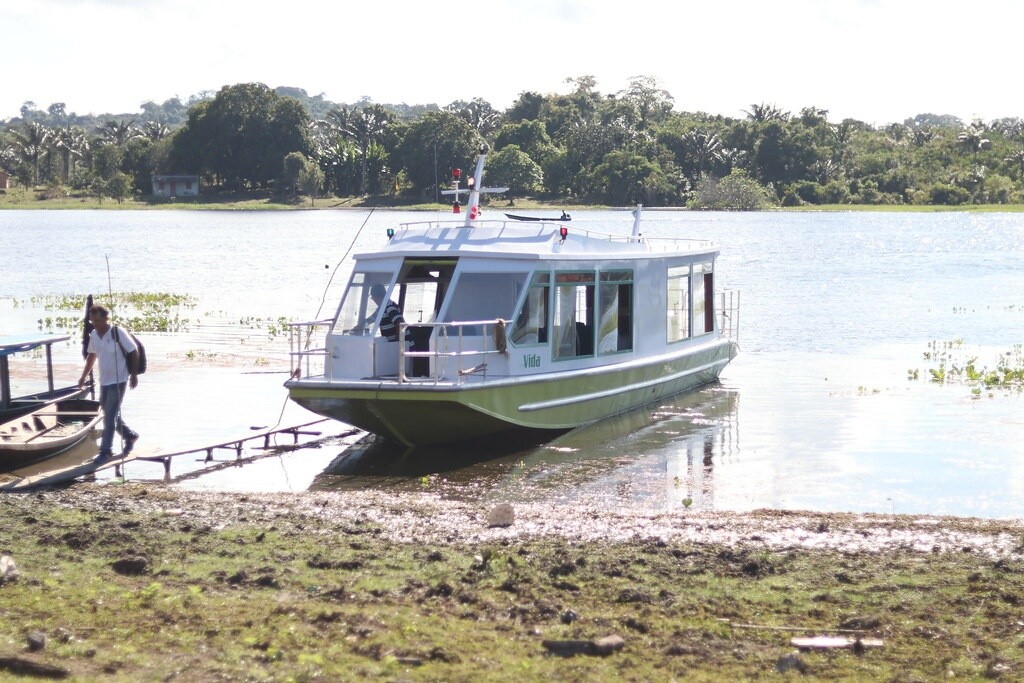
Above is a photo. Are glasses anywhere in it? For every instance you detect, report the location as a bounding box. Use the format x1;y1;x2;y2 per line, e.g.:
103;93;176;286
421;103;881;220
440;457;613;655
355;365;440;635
88;319;94;324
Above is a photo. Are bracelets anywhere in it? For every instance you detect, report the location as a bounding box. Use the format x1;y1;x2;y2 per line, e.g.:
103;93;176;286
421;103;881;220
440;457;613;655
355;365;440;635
81;376;87;381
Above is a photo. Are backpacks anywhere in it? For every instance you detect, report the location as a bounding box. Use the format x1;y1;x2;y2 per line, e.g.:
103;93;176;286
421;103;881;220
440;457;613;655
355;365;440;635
111;323;147;375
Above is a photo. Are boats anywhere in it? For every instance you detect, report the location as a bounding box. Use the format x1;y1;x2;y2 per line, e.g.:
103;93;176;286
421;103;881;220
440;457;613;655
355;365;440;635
0;398;102;463
0;334;96;425
284;153;742;446
503;209;571;222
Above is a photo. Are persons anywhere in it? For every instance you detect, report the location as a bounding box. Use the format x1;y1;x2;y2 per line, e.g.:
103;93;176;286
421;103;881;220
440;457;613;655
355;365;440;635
78;304;140;464
354;284;415;377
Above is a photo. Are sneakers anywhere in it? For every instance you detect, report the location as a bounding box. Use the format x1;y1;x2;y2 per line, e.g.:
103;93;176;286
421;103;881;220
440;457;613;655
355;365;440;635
93;449;113;464
122;430;139;454
